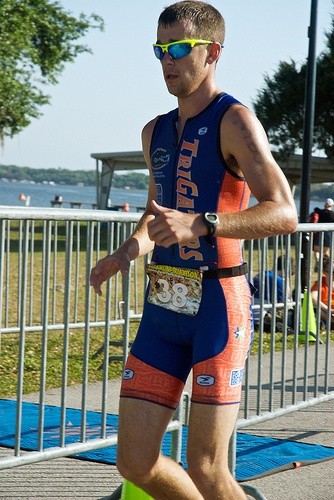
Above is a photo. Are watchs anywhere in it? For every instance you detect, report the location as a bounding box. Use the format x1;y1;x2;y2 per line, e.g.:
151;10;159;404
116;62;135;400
202;211;219;239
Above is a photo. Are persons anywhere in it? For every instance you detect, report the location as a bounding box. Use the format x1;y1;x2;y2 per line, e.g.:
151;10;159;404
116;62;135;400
90;0;298;500
254;199;334;333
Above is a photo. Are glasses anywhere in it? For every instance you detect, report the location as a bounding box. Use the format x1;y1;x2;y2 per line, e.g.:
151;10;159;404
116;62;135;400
153;40;224;60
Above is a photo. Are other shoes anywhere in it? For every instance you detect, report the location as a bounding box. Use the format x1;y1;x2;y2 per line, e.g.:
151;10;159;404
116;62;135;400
315;261;320;272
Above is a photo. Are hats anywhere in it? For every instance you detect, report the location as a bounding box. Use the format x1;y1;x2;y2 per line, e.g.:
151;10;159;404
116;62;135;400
325;198;334;207
